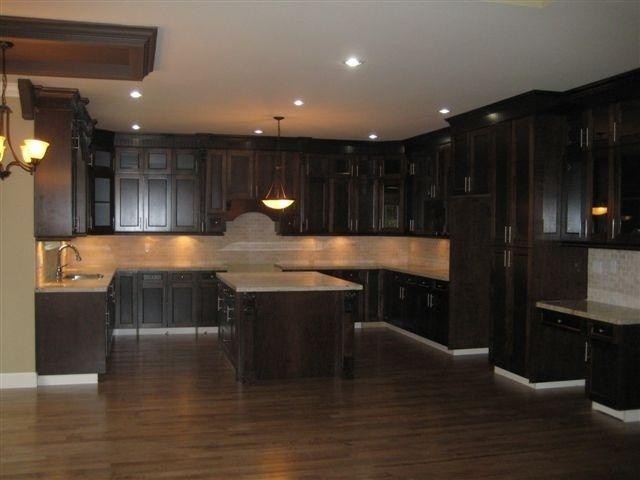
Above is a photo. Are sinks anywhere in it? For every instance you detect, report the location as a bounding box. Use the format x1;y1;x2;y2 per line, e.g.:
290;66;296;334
64;272;104;280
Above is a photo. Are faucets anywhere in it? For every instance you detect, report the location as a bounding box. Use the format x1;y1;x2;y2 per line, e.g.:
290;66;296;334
55;244;82;266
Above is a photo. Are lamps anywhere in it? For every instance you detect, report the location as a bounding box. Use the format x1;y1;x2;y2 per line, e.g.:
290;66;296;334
255;130;265;137
293;99;304;109
437;108;451;116
260;116;295;210
128;90;144;99
130;123;141;135
0;46;49;181
368;134;378;142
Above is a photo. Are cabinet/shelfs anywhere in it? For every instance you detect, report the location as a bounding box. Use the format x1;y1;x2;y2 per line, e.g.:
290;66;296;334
115;147;172;232
381;266;451;345
172;149;203;232
497;113;537;382
34;270;116;374
34;86;89;240
451;128;496;367
537;72;638;248
217;272;347;379
170;270;217;329
254;150;304;233
90;140;114;236
304;148;405;233
335;267;380;322
203;149;254;233
405;139;451;237
537;309;640;410
116;270;170;330
